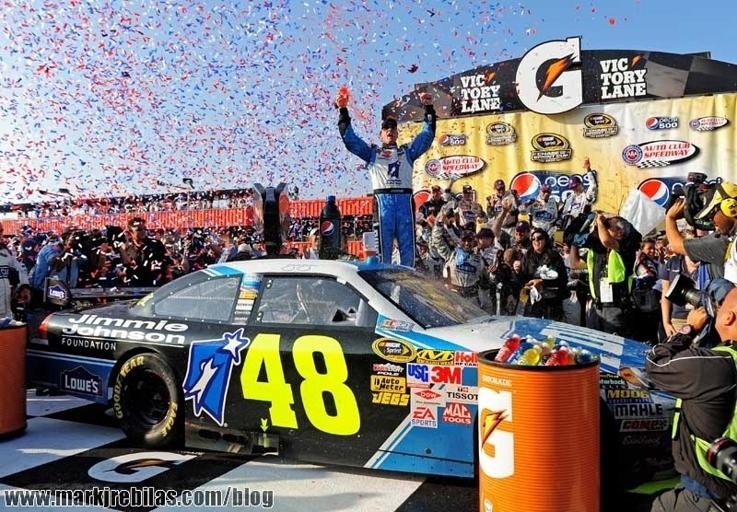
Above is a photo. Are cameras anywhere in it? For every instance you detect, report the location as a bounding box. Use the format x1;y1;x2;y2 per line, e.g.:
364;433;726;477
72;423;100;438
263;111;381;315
703;436;737;512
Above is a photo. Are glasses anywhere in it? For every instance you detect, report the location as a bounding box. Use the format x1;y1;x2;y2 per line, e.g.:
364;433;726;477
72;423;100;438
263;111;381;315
531;235;544;240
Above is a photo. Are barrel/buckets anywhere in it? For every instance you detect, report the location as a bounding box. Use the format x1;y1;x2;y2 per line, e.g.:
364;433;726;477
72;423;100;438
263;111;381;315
0;319;28;441
476;348;602;512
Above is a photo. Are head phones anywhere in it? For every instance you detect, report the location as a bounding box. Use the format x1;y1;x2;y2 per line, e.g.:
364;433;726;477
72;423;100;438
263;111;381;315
715;183;737;218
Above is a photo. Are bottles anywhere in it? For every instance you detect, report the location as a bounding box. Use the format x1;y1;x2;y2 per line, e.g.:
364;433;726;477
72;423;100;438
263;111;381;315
319;196;341;259
337;86;348;108
494;334;590;367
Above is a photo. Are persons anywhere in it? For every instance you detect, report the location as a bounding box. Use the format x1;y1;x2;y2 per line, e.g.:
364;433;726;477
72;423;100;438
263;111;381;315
335;88;436;269
1;160;737;512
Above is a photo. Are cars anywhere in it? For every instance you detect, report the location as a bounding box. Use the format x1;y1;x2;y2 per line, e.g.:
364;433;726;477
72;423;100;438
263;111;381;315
22;258;679;496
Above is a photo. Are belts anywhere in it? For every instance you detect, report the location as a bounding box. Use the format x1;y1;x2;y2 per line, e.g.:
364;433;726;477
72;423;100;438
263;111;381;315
595;302;615;308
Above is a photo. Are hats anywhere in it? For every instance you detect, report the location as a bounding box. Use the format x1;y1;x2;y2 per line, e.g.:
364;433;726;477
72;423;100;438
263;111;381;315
382;118;396;129
569;178;580;188
461;228;494;240
463;185;472;193
542;184;551;193
495;180;505;189
693;181;737;220
516;220;529;233
